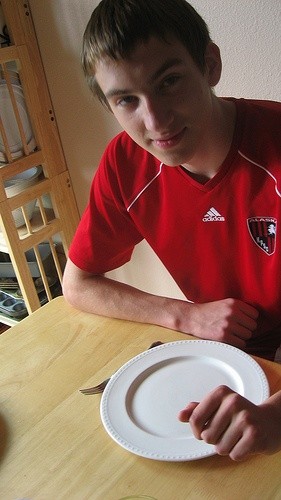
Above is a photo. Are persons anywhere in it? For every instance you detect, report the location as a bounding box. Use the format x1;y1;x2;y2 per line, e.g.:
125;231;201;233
63;0;281;462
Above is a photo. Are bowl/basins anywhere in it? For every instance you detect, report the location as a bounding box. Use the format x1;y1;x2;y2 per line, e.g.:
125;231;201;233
1;164;42;233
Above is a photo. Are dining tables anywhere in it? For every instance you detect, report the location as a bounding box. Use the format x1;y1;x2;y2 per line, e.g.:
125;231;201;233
0;295;281;500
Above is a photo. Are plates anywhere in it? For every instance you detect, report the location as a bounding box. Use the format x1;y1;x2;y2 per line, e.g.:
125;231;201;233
98;339;270;463
0;70;36;163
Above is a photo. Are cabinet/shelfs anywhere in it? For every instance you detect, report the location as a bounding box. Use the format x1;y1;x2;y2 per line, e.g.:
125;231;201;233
0;0;81;326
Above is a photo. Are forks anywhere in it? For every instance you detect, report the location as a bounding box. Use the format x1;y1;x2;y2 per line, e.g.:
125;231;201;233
79;340;165;397
